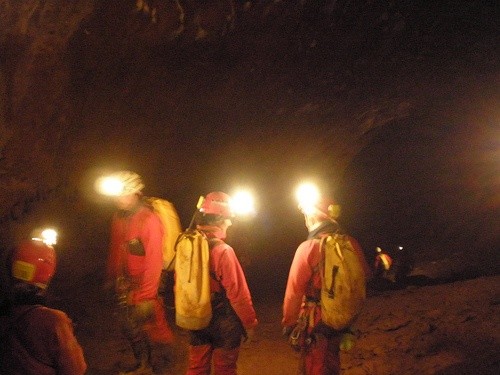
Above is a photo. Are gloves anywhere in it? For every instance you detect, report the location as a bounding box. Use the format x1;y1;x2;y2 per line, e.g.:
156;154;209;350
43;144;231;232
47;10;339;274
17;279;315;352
243;327;254;345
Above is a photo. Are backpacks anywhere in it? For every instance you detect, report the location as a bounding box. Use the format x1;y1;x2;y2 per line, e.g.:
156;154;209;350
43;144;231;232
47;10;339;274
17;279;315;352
379;253;392;272
174;229;220;330
314;234;367;331
147;194;181;266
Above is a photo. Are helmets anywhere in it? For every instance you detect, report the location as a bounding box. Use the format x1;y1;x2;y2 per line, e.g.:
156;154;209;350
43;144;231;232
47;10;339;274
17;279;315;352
299;197;341;224
200;192;236;215
10;238;57;289
100;172;148;197
376;247;381;253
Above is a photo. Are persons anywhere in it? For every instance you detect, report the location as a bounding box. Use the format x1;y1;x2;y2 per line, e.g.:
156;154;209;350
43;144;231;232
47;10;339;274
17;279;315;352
281;190;371;375
102;171;173;374
0;239;87;374
374;247;394;274
187;191;258;375
